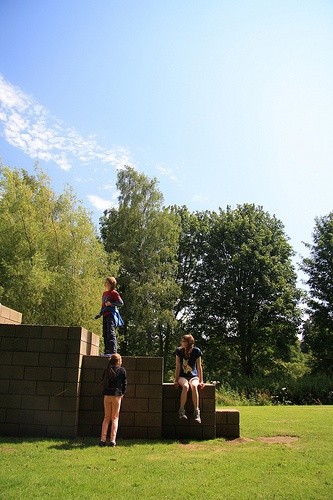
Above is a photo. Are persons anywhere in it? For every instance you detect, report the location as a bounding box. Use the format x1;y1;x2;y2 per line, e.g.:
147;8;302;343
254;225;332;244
100;352;127;447
94;277;124;359
173;334;204;424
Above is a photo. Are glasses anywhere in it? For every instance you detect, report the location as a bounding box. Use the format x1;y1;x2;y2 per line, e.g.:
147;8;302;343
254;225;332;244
181;339;187;343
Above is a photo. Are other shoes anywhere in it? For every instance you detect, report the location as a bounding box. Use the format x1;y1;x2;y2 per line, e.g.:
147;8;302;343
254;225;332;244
102;350;112;357
178;409;187;420
194;409;201;424
108;441;117;447
100;440;106;447
